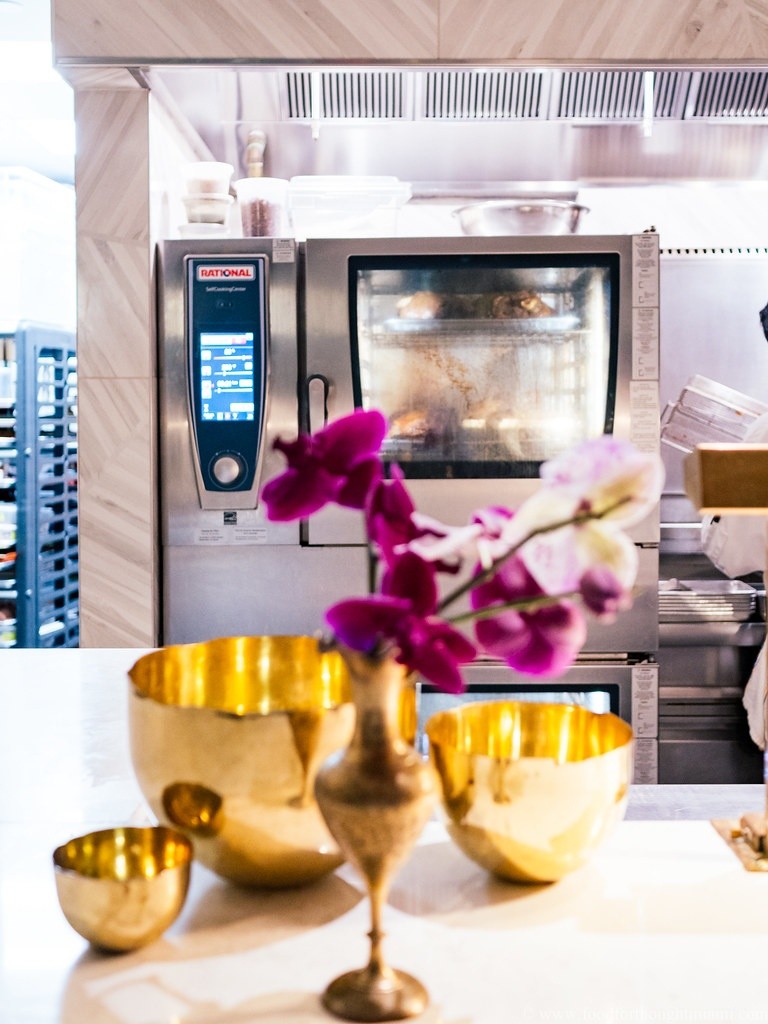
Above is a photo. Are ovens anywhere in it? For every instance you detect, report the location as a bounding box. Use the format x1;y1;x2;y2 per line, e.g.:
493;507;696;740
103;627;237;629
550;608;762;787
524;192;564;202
152;227;663;665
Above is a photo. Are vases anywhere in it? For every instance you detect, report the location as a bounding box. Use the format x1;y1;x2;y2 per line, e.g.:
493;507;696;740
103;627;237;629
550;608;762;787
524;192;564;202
312;627;432;1021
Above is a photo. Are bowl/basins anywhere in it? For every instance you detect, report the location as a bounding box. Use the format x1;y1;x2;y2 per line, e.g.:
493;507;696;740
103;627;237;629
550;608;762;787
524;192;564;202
52;822;194;952
128;630;422;885
424;696;636;886
451;201;588;236
178;162;235;226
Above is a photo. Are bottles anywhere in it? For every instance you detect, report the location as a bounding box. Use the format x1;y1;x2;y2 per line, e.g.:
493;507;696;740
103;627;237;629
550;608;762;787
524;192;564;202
231;175;290;236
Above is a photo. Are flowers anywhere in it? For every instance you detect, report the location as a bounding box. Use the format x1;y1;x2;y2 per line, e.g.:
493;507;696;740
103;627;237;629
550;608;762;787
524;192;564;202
259;410;665;698
